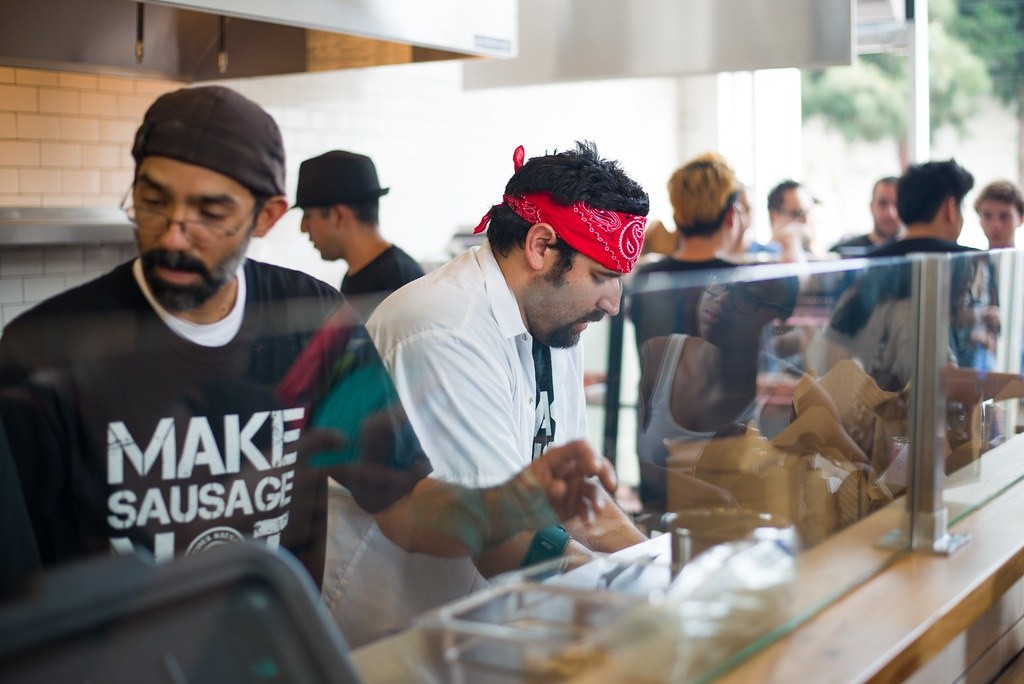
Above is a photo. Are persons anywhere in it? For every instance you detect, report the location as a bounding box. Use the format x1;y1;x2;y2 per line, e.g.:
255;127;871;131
828;177;902;259
637;246;878;580
319;139;650;648
971;180;1024;373
790;157;1000;443
767;179;824;262
285;151;424;325
625;150;809;533
0;85;618;596
849;259;1024;417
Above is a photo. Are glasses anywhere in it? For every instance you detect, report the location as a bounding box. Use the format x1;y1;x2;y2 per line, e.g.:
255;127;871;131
120;179;258;249
702;273;793;317
775;206;810;220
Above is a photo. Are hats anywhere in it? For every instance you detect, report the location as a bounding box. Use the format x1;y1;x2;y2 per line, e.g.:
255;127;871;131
284;152;387;209
132;85;284;196
